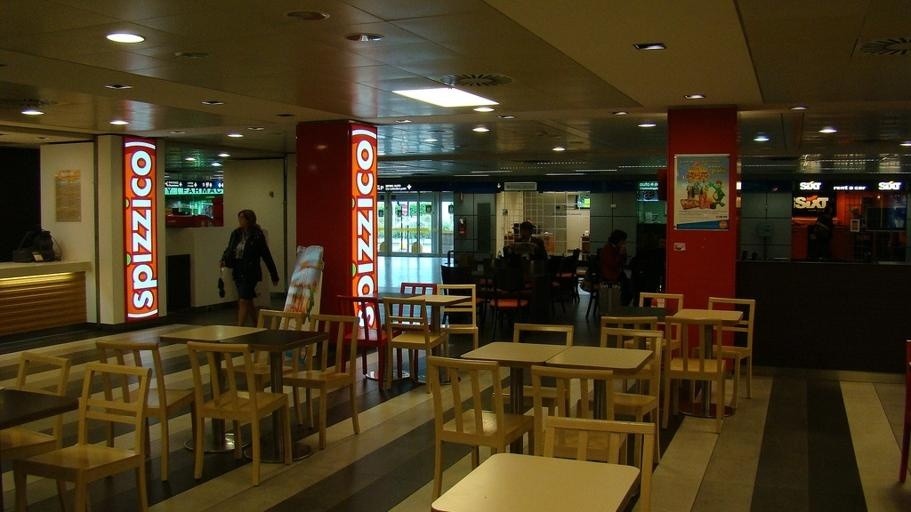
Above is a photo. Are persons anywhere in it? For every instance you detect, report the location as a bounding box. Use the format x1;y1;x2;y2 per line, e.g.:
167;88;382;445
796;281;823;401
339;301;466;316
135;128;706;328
218;210;280;327
633;232;665;307
493;245;531;329
600;230;639;307
515;222;545;316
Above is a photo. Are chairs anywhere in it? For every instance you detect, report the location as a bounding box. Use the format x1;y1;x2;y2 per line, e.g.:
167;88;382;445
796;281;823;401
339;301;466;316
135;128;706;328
707;298;756;409
430;356;535;505
531;364;632;464
544;416;655;511
428;251;745;431
13;363;152;510
338;282;479;390
96;310;361;486
600;328;664;465
1;351;79;512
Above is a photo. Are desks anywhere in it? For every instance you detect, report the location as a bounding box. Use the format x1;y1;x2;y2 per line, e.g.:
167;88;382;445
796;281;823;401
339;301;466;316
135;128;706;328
431;453;641;511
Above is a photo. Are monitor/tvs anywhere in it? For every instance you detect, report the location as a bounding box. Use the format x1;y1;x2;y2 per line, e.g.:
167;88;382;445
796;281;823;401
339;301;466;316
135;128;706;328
793;189;837;218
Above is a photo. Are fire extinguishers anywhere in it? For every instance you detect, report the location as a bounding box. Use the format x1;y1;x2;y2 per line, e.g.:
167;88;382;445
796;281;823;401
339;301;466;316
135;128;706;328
458;219;465;236
